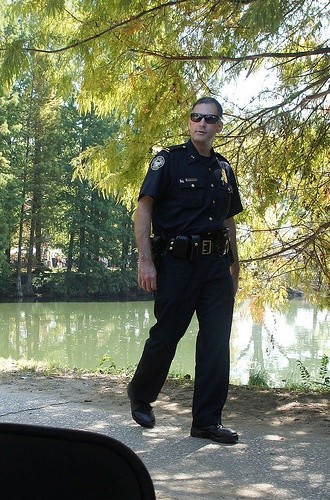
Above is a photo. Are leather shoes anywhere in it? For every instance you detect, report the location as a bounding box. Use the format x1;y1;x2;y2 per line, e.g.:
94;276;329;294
128;385;154;428
192;422;241;443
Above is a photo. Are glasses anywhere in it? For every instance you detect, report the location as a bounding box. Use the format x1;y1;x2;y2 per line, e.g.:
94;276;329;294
190;112;221;124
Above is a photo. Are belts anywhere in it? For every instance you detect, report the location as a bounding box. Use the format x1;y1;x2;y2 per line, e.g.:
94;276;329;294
156;231;234;261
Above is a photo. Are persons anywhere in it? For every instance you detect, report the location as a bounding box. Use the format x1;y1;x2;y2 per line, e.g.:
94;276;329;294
127;98;245;444
